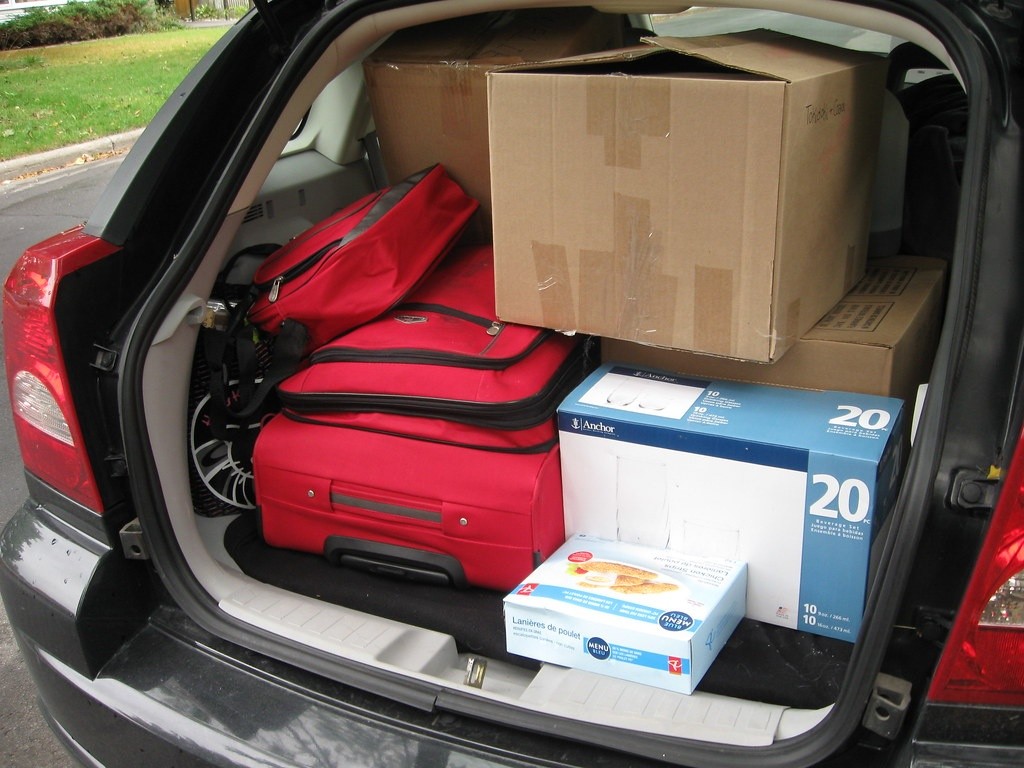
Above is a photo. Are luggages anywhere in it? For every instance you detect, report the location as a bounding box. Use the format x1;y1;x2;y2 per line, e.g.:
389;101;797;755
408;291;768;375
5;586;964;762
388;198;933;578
251;246;603;591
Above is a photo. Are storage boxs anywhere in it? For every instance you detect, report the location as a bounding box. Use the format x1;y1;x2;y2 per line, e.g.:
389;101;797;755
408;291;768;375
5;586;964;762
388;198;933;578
600;253;951;452
503;531;748;697
556;364;907;644
361;10;626;245
485;27;894;367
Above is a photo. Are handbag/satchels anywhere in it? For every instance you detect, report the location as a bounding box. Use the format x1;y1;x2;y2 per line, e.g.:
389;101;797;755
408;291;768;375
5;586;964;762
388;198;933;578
247;164;480;363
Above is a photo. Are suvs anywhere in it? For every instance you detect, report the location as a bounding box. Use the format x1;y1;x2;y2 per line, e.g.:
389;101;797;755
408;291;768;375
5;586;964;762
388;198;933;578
0;0;1024;768
